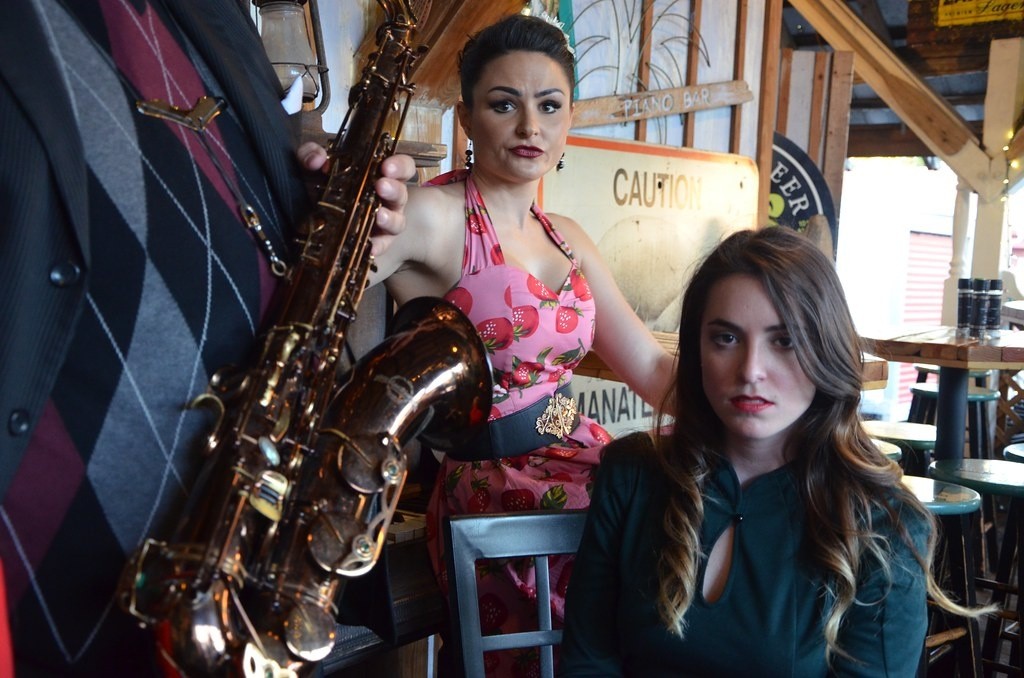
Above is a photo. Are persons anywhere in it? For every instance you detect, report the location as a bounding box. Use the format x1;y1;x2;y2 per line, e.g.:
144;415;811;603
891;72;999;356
0;0;418;678
365;14;677;678
556;225;999;678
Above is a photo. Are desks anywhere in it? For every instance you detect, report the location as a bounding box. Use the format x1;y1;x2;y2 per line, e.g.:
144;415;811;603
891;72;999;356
855;301;1024;460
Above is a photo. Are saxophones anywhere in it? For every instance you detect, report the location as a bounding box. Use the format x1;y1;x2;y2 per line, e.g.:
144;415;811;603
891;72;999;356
116;0;497;678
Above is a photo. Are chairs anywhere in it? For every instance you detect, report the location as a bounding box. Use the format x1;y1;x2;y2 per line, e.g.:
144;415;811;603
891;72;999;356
446;507;587;678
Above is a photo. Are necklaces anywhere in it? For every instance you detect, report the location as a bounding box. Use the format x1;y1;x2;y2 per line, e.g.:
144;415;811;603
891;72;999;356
133;89;288;279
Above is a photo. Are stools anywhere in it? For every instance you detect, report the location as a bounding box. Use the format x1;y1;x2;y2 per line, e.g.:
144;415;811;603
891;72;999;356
861;362;1024;678
